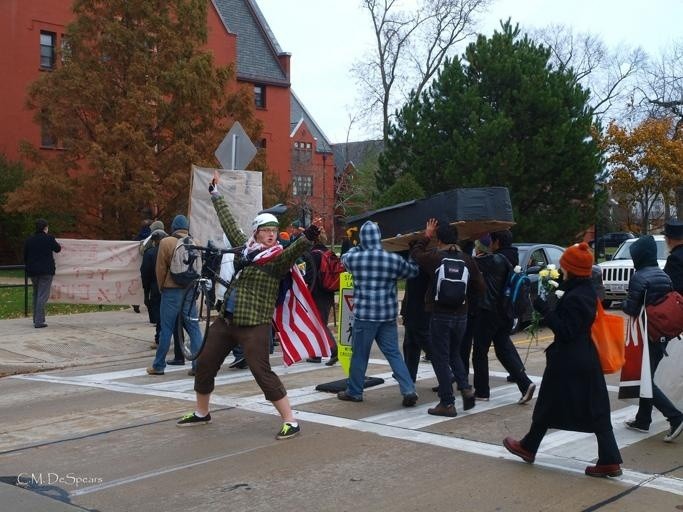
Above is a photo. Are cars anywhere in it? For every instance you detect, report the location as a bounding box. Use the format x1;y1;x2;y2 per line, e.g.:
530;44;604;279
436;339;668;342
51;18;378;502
508;242;605;338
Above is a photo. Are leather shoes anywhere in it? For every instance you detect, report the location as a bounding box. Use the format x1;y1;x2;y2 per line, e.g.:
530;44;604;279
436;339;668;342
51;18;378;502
585;464;622;477
503;437;535;463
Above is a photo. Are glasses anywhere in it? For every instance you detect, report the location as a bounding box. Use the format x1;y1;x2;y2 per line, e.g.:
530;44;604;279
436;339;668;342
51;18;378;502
257;227;279;233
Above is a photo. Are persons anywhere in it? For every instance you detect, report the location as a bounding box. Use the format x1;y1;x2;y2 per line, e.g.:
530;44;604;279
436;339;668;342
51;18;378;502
132;215;341;376
625;236;683;443
658;218;683;299
175;167;328;440
399;215;536;419
22;217;61;328
500;238;623;479
335;220;419;408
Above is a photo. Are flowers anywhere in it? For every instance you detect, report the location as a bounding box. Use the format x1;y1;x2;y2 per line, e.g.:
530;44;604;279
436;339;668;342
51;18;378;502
513;260;566;347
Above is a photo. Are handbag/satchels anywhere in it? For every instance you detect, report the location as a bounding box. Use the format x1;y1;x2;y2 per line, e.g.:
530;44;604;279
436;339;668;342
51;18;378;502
591;314;626;372
642;291;683;346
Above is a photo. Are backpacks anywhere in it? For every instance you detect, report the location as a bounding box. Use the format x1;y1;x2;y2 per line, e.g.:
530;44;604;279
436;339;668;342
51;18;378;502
487;253;533;319
275;271;293;306
433;245;471;309
311;249;344;292
169;233;202;286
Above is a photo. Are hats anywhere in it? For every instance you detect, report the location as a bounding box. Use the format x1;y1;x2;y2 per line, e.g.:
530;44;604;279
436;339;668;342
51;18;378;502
150;230;168;241
278;232;290;245
660;221;683;237
474;232;493;254
251;212;279;235
559;242;593;277
171;215;190;232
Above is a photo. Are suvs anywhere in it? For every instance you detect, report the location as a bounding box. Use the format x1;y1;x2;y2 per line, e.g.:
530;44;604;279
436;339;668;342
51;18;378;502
593;233;683;310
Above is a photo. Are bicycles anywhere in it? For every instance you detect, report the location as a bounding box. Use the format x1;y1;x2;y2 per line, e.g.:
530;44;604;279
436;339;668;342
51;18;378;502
175;239;246;363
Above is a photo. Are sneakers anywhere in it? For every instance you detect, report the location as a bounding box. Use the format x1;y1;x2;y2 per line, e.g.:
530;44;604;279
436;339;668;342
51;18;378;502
35;324;48;328
664;415;683;441
175;412;212;427
276;422;300;440
625;420;650;432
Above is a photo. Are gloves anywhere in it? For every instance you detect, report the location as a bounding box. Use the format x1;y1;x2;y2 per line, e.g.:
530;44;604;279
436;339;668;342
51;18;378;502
533;295;548;318
208;180;218;194
303;224;321;240
